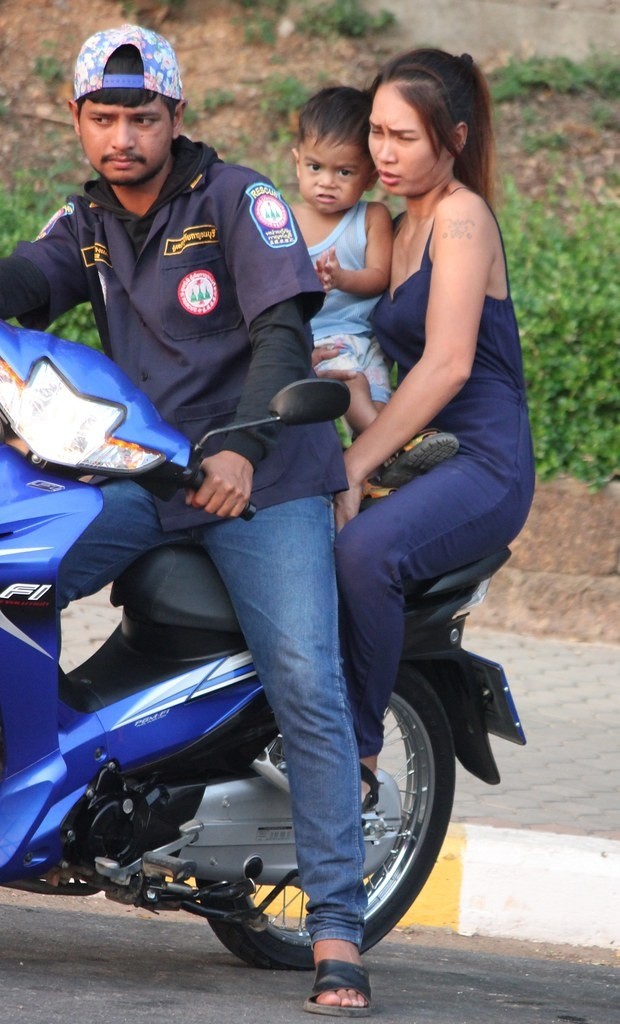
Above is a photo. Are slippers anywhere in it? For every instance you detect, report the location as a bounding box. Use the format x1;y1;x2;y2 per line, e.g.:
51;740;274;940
303;959;372;1018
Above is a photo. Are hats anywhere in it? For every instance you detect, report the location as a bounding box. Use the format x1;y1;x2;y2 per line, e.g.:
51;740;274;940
72;23;182;102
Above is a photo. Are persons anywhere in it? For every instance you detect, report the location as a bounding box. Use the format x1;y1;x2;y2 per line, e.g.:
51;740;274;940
0;24;371;1017
287;86;460;486
311;48;535;808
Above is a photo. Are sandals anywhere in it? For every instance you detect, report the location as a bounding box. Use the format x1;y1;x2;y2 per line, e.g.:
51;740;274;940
368;428;460;488
360;763;380;813
360;481;396;509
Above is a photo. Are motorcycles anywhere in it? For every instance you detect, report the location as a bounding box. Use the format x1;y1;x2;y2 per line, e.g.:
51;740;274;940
0;319;526;971
